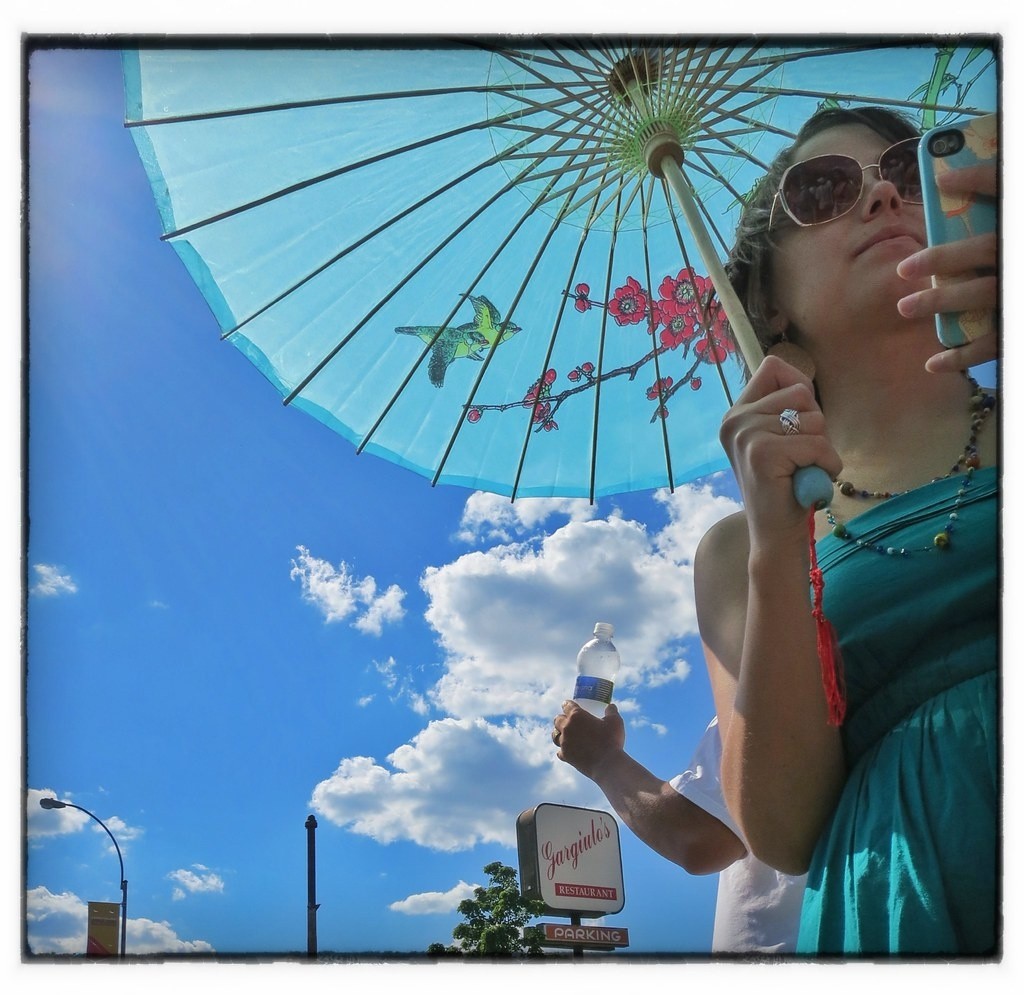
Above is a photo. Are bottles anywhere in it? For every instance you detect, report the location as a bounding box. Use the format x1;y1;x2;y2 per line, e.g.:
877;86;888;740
571;622;621;719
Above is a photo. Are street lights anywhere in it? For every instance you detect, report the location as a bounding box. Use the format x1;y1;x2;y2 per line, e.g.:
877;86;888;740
39;799;127;956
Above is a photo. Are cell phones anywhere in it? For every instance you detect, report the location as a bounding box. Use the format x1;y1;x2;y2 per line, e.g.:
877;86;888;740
918;112;998;349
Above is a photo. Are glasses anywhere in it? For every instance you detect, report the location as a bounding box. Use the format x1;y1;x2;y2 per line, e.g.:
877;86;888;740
767;136;927;233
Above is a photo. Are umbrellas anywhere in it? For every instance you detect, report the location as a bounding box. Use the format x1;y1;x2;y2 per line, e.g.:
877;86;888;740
120;48;1004;503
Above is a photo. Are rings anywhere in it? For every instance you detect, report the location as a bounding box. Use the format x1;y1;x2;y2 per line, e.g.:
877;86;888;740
779;408;801;435
552;729;561;747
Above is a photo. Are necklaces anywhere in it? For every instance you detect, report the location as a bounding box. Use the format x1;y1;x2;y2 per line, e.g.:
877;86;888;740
824;373;991;557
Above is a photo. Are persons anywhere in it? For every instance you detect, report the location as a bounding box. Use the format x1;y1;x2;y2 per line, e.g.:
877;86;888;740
691;106;1000;955
551;620;799;955
